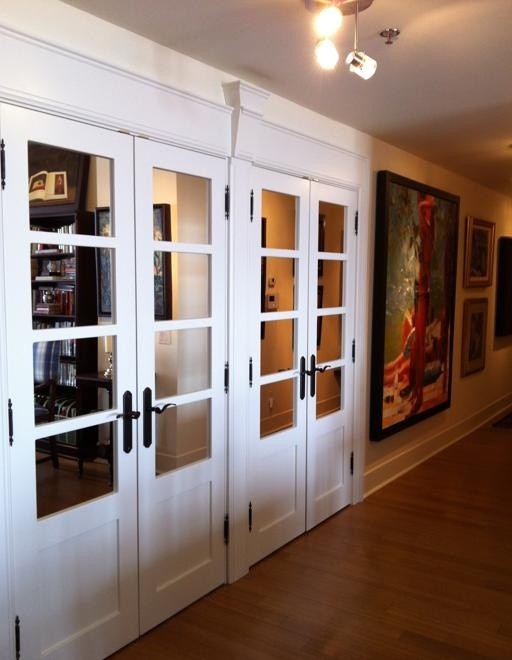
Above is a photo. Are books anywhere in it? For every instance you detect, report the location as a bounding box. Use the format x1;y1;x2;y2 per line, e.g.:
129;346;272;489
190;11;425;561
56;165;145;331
29;222;78;389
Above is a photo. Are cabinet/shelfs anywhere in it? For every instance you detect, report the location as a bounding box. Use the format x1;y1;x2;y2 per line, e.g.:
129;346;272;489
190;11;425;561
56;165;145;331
30;212;99;461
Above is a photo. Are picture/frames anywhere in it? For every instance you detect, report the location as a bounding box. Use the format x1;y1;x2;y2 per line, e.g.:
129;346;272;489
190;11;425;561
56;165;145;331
369;167;460;443
460;297;488;376
29;142;90;212
462;215;497;288
95;206;172;321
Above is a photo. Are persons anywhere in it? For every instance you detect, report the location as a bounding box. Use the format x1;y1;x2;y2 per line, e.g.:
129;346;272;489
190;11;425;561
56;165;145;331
404;190;439;420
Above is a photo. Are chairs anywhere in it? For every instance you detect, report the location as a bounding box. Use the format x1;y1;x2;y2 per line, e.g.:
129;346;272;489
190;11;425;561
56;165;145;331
29;341;59;468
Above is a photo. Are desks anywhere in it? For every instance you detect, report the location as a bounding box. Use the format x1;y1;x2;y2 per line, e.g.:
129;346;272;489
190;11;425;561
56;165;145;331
74;371;112;486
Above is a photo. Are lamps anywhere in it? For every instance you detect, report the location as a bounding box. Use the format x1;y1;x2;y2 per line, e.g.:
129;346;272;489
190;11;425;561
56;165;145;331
104;335;113;380
303;0;378;80
